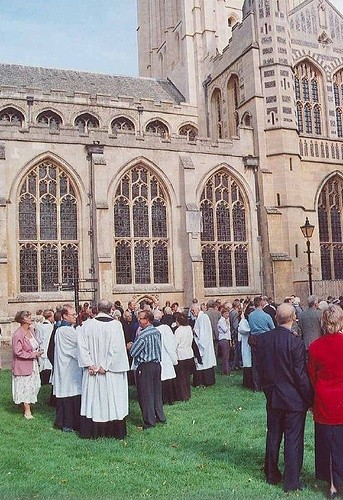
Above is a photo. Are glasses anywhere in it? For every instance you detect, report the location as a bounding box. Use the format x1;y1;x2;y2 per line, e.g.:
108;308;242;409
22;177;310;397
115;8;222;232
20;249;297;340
68;313;78;317
139;318;146;320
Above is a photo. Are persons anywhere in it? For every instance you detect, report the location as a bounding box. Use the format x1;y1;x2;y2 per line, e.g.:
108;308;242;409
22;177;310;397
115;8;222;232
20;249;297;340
230;301;243;370
52;307;83;433
300;296;322;354
130;309;166;430
217;311;232;376
256;302;312;493
12;312;43;419
190;303;217;388
76;301;130;439
307;305;343;496
29;295;343;405
260;295;276;325
249;296;275;393
174;315;193;402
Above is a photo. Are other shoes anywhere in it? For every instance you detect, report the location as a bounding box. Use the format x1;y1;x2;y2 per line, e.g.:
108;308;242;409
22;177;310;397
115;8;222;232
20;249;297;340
137;424;148;429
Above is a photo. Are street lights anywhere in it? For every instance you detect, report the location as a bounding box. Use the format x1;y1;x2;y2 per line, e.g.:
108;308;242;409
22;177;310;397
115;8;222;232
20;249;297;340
300;217;315;294
